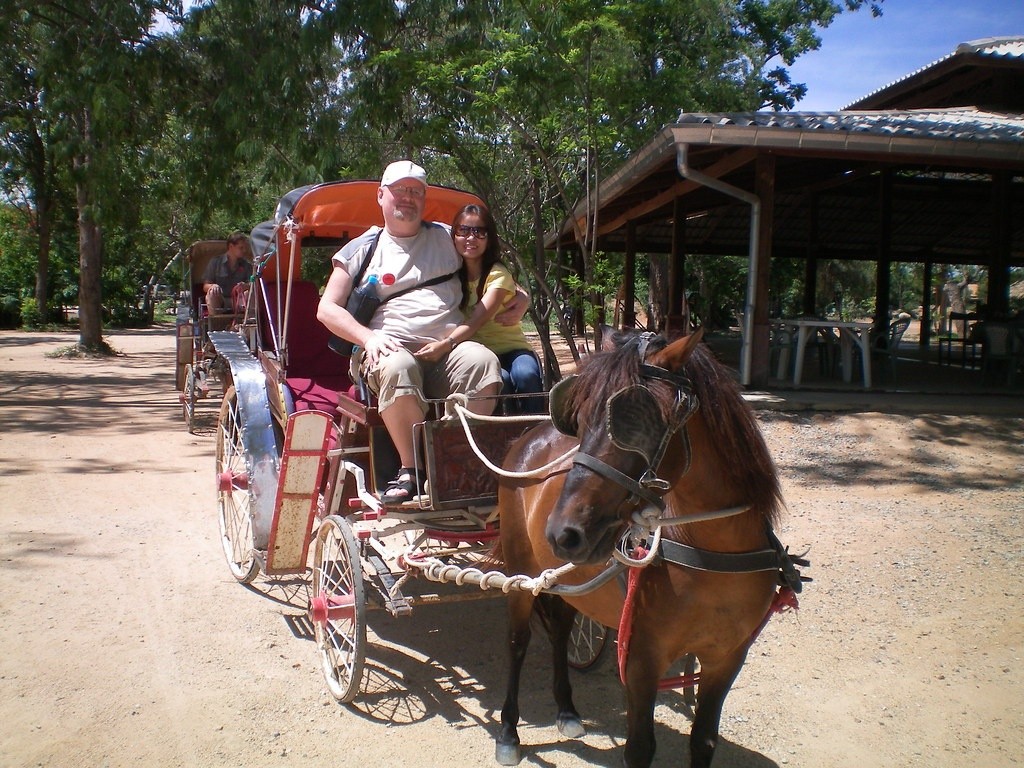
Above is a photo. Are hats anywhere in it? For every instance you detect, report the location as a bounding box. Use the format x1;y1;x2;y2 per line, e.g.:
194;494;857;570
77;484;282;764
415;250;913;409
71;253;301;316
381;160;428;188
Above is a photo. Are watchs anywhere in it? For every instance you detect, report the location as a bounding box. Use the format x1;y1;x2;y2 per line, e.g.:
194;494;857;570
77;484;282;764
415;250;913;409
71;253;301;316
448;336;457;349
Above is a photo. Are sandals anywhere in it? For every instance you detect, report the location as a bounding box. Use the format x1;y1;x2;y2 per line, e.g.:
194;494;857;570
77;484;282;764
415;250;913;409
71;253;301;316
382;468;428;504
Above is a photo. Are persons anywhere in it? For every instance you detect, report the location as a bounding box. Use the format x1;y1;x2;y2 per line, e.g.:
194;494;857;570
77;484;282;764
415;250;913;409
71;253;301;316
202;232;253;316
316;160;544;506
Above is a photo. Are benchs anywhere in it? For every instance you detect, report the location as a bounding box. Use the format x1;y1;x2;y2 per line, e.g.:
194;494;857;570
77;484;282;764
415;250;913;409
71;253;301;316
194;282;209;325
263;280;387;460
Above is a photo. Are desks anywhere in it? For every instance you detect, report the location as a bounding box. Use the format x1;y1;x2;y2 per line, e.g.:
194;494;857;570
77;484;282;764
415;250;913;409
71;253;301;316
769;317;875;389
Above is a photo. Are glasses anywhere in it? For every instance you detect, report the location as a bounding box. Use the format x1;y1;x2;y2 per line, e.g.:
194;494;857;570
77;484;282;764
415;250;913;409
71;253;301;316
383;185;425;198
454;224;489;239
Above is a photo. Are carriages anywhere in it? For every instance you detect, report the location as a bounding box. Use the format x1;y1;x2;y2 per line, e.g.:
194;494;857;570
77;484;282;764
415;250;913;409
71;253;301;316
210;181;816;768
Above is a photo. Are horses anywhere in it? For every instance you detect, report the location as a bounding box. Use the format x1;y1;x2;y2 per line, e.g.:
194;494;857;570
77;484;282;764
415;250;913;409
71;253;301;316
495;324;782;767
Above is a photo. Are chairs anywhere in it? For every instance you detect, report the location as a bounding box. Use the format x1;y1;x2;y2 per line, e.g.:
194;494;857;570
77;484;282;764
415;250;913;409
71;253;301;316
853;317;911;386
789;315;835;378
736;313;796;382
939;312;977;371
981;321;1024;389
